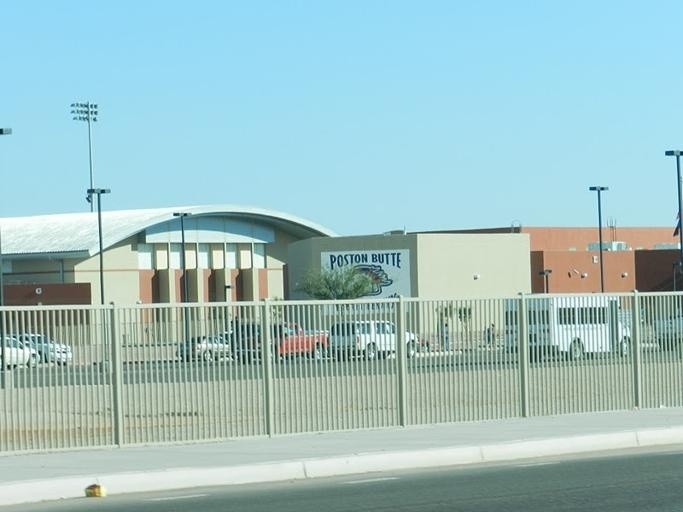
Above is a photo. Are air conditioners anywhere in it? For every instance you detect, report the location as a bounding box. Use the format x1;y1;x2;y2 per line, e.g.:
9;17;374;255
611;240;626;251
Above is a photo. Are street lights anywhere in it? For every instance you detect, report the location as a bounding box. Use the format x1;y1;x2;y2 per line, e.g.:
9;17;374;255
85;187;113;306
663;147;682;287
538;268;553;295
67;99;101;212
172;210;192;342
669;261;680;291
0;127;13;135
587;185;610;294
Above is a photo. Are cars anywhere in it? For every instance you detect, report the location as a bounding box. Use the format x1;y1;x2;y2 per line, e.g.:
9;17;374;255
175;313;423;369
650;307;681;352
0;335;41;369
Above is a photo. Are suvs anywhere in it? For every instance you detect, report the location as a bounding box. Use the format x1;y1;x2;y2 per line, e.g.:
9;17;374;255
10;332;73;368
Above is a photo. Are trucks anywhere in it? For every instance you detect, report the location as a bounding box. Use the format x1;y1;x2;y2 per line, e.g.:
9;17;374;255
501;290;634;364
